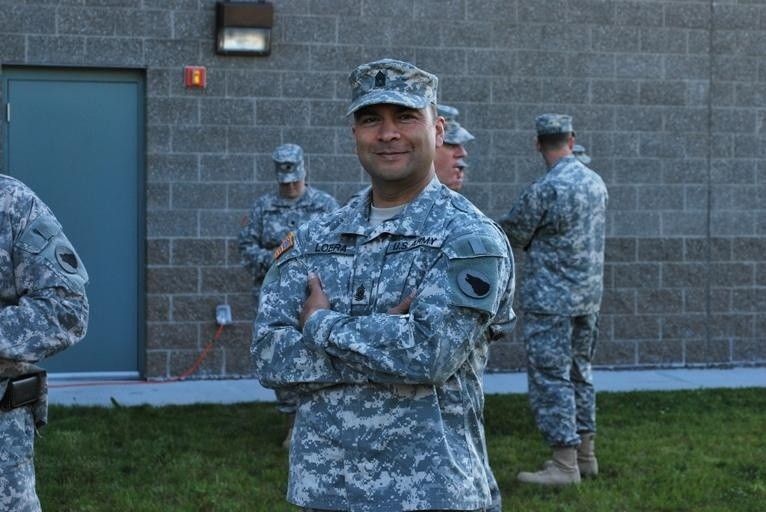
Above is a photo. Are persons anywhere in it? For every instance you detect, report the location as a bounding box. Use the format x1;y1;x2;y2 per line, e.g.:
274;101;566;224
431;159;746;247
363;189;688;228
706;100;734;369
500;113;607;488
249;58;517;512
567;144;591;167
236;143;340;453
451;156;468;192
0;173;89;512
431;102;474;187
436;105;459;121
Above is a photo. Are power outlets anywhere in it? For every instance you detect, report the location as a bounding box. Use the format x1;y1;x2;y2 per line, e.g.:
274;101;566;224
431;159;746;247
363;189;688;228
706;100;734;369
215;302;233;329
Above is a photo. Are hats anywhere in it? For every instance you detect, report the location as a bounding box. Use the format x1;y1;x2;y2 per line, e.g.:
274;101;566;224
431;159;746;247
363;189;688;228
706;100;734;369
344;59;475;168
573;145;592;164
272;143;307;184
535;114;577;136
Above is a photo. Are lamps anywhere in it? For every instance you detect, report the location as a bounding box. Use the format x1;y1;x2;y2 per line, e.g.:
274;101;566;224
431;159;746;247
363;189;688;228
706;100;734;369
214;1;276;55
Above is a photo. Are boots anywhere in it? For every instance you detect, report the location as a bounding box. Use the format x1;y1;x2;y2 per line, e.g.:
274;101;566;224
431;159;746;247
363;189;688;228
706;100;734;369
517;445;582;487
543;433;598;480
282;413;295;450
